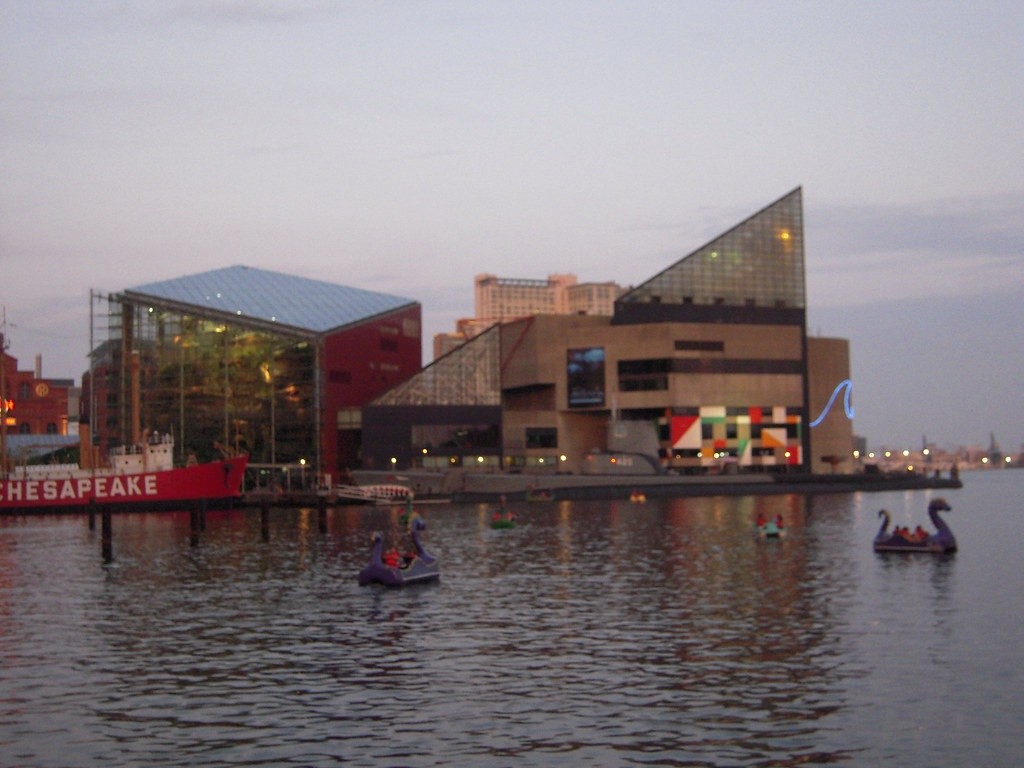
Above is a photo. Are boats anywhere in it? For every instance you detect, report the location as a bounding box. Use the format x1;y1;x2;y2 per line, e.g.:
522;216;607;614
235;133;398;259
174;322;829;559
490;493;516;528
360;518;442;585
0;429;250;512
399;496;422;523
872;498;958;556
751;520;787;539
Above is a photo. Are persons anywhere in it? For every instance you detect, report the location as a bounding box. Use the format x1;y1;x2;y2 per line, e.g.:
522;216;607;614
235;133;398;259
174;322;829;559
385;547;418;568
756;514;781;528
893;525;927;542
